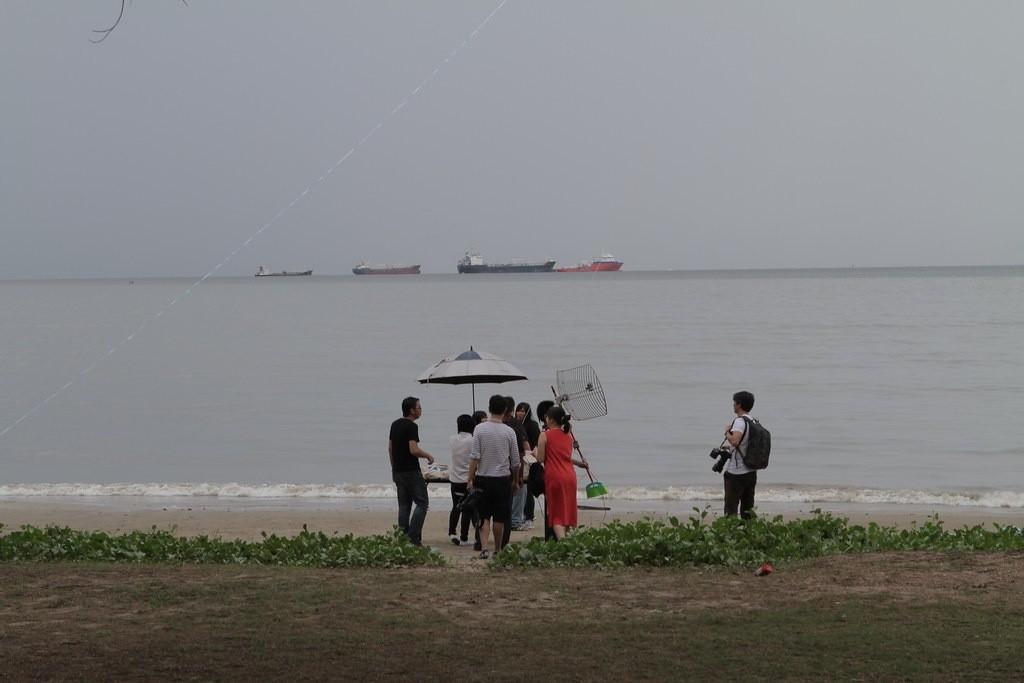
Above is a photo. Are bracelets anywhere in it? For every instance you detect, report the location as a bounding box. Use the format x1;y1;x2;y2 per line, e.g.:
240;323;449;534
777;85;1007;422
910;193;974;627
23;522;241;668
724;430;729;437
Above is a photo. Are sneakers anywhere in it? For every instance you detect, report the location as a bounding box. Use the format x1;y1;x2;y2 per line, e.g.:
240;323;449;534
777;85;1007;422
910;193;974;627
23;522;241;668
451;534;468;546
511;520;534;531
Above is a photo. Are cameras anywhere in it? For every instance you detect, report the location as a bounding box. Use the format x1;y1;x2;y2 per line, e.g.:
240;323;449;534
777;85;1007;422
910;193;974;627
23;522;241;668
709;448;731;473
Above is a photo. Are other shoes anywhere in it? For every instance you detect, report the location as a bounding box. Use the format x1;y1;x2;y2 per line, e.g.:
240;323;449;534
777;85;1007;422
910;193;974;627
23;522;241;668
473;544;481;550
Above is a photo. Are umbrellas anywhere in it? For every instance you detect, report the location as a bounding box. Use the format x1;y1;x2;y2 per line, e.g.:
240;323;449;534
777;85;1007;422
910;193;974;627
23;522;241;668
413;346;528;411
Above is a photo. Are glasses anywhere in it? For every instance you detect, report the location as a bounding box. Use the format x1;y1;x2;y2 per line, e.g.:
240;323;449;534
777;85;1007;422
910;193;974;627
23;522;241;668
415;407;421;410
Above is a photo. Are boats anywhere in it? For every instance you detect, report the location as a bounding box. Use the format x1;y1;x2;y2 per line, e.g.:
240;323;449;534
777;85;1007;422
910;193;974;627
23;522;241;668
254;266;315;277
456;252;557;272
351;263;421;274
555;254;624;272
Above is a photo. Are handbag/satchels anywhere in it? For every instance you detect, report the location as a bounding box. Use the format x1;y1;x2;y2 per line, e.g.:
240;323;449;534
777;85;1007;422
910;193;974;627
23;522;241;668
528;462;544;498
461;489;486;521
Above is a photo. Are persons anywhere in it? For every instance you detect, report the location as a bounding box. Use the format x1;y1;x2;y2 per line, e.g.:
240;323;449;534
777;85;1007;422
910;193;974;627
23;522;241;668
720;391;757;519
448;394;578;559
388;396;435;548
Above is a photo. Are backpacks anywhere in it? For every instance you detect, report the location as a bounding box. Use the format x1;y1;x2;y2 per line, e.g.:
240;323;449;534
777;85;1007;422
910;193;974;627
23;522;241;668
729;416;771;470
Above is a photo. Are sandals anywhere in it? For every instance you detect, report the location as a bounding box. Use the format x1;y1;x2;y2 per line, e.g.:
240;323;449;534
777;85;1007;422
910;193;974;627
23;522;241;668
478;549;489;559
